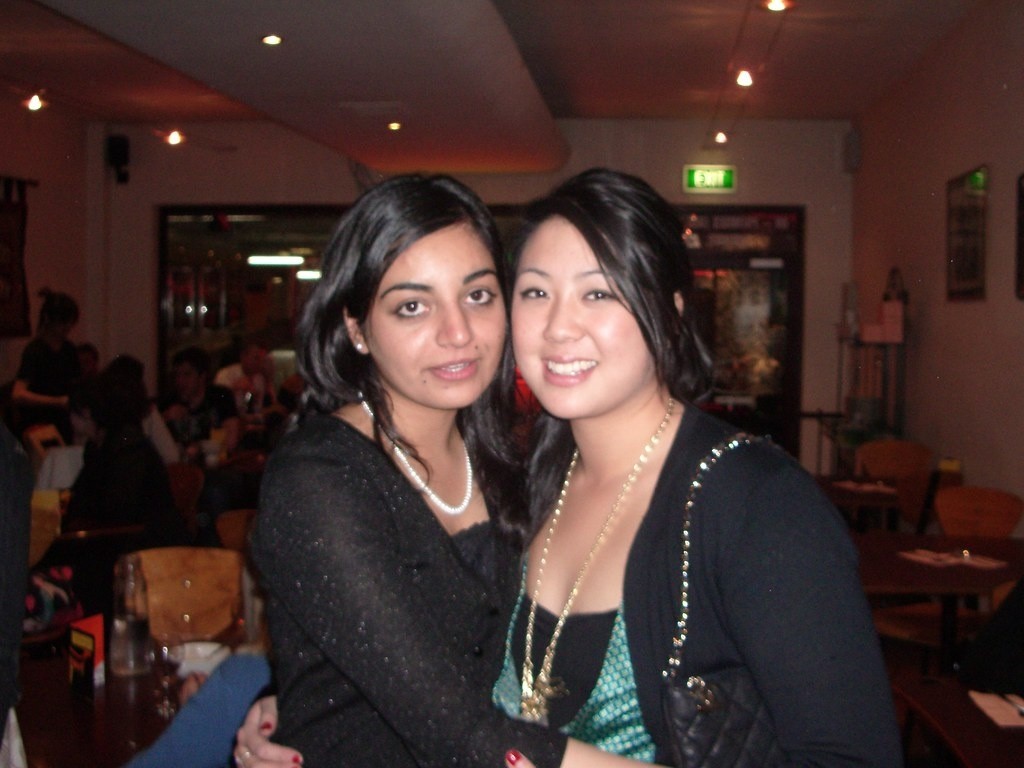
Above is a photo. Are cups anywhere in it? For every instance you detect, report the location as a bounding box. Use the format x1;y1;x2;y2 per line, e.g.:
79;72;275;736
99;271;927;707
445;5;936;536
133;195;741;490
166;641;230;721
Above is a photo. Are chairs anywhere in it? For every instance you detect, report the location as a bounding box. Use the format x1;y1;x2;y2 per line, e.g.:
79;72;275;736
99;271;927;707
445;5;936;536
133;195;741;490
215;509;256;551
169;461;205;543
114;546;244;644
872;562;1024;674
934;487;1024;540
845;439;940;535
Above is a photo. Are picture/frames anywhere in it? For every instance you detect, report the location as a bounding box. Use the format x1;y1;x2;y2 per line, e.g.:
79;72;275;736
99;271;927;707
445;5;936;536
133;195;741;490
945;165;987;299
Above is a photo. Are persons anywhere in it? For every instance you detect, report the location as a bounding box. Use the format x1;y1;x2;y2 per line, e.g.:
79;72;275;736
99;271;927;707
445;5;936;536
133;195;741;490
232;168;909;768
246;173;669;768
0;423;31;768
952;577;1024;694
13;284;334;520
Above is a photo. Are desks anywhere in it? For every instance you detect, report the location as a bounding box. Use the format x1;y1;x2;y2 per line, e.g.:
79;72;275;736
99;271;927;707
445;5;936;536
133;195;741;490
18;638;246;768
892;671;1024;768
853;534;1024;599
203;449;267;506
814;474;898;532
56;516;146;614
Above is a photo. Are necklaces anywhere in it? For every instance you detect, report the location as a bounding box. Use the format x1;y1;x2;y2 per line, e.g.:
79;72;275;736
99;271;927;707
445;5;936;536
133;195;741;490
359;392;472;515
519;395;676;726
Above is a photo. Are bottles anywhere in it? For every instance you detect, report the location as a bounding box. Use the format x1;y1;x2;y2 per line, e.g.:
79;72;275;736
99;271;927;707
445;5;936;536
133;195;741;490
108;553;150;710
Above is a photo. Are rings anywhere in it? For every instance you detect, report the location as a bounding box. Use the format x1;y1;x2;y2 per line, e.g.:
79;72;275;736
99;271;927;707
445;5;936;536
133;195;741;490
235;751;254;762
